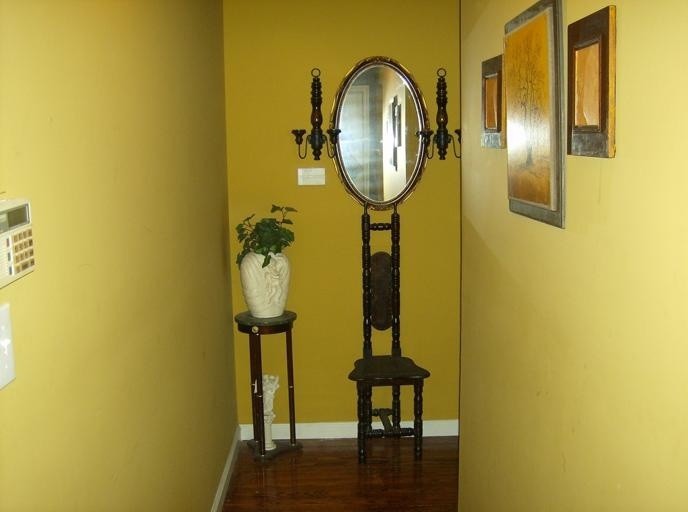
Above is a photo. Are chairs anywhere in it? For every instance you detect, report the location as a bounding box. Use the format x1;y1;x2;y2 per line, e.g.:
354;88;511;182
347;212;430;464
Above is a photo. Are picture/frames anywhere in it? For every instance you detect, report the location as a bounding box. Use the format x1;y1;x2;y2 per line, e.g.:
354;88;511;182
480;54;507;149
567;4;617;159
504;0;568;229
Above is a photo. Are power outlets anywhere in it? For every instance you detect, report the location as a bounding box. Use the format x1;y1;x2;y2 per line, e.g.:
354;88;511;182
0;303;16;391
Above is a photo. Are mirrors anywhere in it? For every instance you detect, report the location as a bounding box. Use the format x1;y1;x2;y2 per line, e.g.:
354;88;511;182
330;55;431;210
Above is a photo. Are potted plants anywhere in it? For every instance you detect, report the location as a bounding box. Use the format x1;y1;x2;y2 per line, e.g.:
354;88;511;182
235;204;298;318
